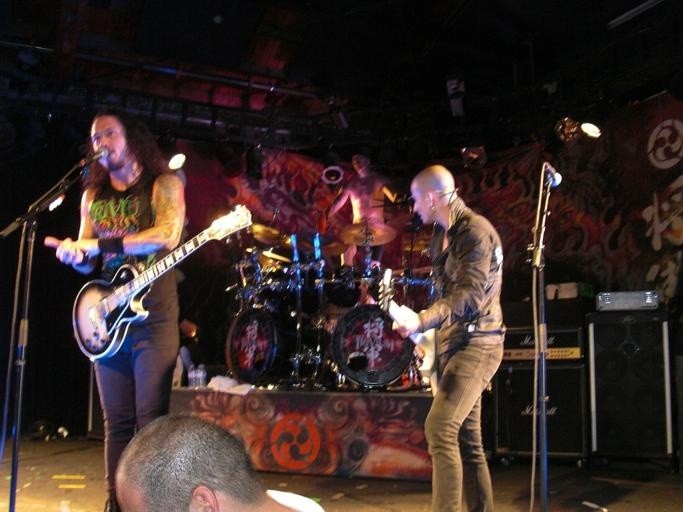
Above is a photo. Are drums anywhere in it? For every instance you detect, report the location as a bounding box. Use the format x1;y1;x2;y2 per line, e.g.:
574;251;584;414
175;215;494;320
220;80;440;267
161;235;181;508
325;265;363;308
265;268;311;310
226;308;312;387
333;303;415;388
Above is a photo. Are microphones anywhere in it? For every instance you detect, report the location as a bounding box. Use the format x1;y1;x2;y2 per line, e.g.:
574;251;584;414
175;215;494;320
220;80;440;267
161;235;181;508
544;163;563;186
80;147;108;166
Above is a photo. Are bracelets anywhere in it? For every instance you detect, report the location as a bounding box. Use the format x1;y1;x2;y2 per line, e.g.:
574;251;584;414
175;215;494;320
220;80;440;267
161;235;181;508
97;235;125;255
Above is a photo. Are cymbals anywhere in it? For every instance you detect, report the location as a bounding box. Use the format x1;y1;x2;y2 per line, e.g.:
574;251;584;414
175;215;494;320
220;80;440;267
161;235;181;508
249;223;279;239
246;246;291;263
339;221;397;246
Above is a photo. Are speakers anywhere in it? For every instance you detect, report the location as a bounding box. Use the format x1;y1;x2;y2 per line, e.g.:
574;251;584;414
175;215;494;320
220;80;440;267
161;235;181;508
495;366;583;457
586;317;672;460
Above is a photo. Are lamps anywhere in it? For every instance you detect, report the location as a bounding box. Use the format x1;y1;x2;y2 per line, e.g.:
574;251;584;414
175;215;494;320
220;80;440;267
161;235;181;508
154;133;188;172
576;101;614;140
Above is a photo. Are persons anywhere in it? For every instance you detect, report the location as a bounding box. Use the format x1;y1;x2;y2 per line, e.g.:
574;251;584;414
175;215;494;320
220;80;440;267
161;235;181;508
389;163;507;511
322;273;376;336
112;411;326;512
324;150;407;263
43;105;187;512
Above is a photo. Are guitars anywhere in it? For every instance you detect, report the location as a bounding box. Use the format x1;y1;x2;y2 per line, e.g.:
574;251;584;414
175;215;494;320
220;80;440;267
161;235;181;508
377;269;438;397
73;206;253;361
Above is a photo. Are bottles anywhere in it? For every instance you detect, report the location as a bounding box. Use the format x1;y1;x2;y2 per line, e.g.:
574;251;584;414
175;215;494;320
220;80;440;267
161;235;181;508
187;364;195;388
196;363;207;386
